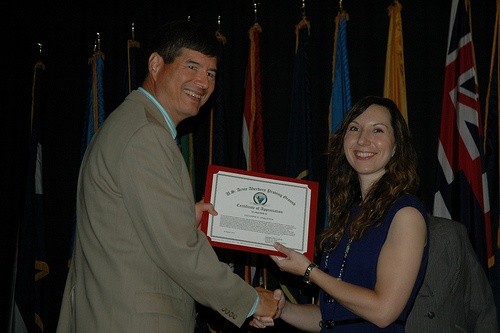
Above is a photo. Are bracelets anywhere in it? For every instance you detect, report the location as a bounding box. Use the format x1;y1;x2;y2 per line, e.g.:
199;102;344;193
302;263;318;287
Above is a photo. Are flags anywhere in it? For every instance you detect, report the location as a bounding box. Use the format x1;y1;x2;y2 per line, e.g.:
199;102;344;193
284;18;317;180
324;13;352;230
85;50;105;146
127;38;141;93
383;2;409;127
5;59;51;333
174;120;195;199
237;21;272;287
432;0;495;289
205;30;235;263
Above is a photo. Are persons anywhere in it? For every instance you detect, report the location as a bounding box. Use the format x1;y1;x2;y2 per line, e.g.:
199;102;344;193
405;217;499;333
55;21;279;333
248;96;430;333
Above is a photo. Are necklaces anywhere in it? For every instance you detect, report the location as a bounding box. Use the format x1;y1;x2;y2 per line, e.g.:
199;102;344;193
322;227;355;304
